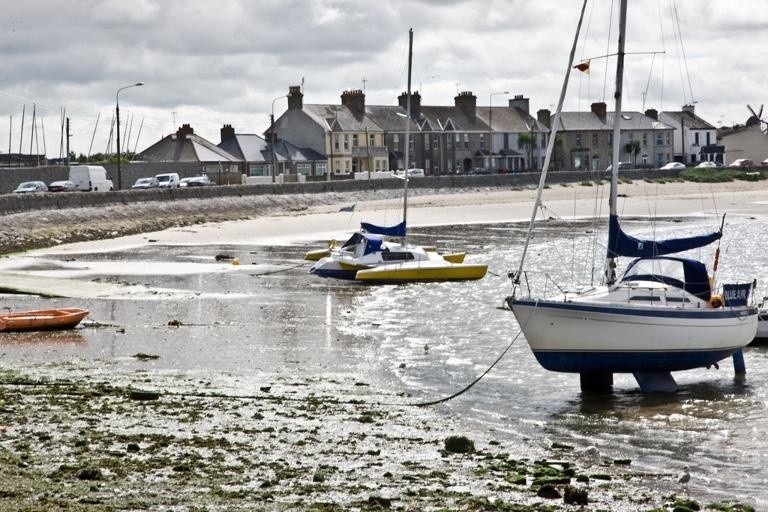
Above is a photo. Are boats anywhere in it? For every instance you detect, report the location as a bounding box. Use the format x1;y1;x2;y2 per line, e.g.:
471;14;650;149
0;308;90;332
755;307;768;339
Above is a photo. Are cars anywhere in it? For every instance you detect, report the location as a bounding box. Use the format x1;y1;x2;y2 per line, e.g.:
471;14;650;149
12;181;79;194
131;172;211;189
729;158;755;167
695;161;717;168
406;169;425;178
606;162;632;170
660;162;687;170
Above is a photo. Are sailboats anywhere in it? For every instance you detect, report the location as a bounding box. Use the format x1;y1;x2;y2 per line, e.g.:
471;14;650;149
505;0;759;373
304;28;488;284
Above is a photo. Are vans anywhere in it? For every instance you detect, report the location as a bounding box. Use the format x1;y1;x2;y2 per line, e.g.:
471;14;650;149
70;165;114;192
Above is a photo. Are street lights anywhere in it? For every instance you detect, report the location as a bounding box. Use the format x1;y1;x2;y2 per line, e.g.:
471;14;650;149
489;92;510;173
116;82;144;190
271;94;292;182
